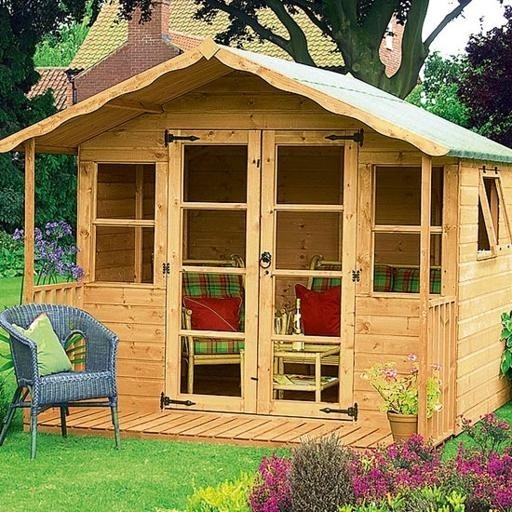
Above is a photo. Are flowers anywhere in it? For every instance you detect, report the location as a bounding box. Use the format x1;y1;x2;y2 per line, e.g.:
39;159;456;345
357;350;443;418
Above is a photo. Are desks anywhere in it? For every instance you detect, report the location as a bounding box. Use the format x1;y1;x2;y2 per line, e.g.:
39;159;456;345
273;341;342;403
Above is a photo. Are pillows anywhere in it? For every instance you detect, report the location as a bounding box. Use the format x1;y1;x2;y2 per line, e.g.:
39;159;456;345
292;283;350;334
181;295;243;333
7;311;75;377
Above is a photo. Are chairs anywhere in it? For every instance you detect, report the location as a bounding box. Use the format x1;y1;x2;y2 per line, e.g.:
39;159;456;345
0;303;128;462
174;253;253;394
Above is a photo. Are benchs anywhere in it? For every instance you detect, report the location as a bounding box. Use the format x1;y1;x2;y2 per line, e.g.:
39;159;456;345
271;253;442;404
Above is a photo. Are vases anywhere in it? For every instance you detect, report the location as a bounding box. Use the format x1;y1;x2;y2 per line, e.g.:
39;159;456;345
384;408;434;447
